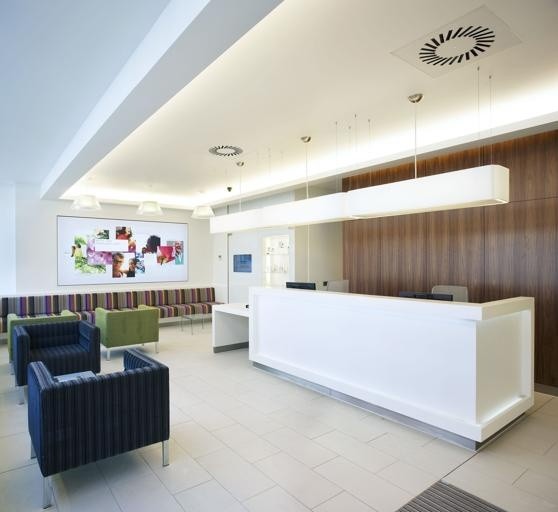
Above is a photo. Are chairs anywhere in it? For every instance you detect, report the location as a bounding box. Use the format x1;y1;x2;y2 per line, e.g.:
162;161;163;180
7;304;171;509
325;278;350;292
431;282;470;302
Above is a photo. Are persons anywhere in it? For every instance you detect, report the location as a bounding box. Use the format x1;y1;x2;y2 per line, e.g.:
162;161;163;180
126;257;135;276
116;227;127;236
112;252;124;278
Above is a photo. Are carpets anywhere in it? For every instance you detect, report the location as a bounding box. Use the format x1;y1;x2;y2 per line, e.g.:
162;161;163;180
390;479;508;512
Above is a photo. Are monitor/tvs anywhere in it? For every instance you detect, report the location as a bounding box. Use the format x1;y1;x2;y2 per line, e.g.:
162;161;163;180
398;291;453;301
286;282;316;290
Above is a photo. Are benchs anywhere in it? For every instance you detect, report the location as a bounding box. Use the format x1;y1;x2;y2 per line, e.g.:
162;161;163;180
1;287;224;343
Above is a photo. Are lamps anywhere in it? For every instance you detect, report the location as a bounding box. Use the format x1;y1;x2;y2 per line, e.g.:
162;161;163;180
70;193;215;220
210;66;510;235
